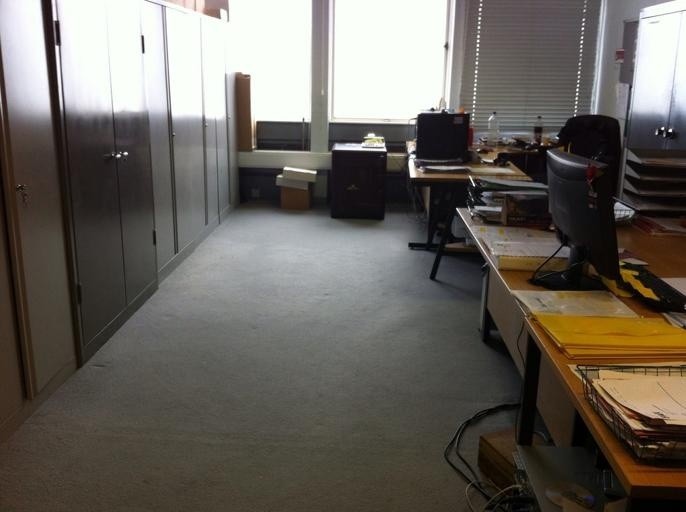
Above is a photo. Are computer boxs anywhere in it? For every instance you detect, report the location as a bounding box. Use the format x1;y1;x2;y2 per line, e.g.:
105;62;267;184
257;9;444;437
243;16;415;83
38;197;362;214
417;113;470;163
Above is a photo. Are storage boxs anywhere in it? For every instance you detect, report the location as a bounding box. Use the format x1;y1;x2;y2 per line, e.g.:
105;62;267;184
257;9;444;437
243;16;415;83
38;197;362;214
280;186;312;210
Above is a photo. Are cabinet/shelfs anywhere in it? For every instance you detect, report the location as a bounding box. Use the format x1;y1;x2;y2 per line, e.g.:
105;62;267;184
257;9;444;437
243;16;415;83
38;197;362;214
329;141;388;221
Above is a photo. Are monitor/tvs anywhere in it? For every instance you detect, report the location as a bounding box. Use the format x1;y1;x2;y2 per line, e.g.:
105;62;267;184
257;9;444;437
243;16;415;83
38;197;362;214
525;147;620;294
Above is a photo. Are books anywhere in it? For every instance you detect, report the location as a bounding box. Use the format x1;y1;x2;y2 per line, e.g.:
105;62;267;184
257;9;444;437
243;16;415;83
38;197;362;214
413;158;470;174
507;289;686;465
466;175;573;272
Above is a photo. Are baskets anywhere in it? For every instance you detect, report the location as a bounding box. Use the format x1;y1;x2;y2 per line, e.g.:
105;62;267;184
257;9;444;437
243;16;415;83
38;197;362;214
571;362;686;468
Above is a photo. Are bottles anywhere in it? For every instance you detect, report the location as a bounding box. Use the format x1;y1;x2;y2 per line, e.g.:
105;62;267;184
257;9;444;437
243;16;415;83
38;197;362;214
534;115;542;144
486;111;499;147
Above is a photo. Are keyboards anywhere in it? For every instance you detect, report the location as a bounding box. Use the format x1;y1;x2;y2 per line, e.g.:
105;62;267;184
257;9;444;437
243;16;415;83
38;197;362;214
617;264;686;314
